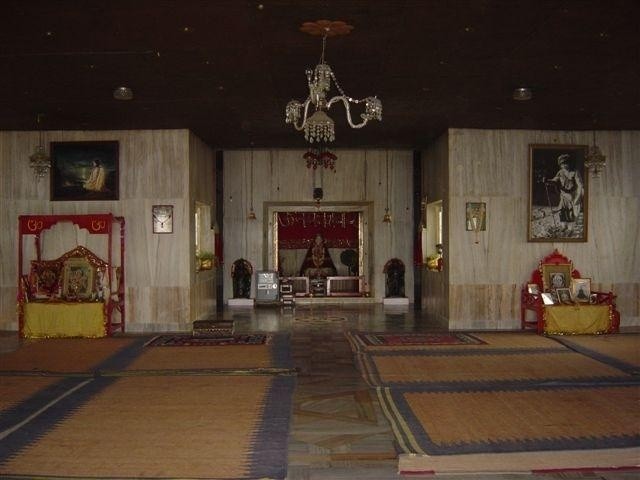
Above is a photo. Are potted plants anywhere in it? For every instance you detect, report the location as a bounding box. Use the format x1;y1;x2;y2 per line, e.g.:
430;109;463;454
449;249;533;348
199;251;215;270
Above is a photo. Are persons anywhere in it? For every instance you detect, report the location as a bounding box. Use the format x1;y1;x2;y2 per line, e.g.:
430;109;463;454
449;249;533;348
81;158;106;192
300;231;338;277
558;290;573;305
541;153;585;237
545;273;567;304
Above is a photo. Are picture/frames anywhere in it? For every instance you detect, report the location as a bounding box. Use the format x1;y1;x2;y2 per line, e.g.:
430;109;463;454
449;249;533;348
466;202;486;231
572;278;591;303
542;264;572;301
62;257;96;300
50;140;119;202
527;143;588;242
152;204;174;234
556;288;572;304
29;260;62;300
527;282;542;299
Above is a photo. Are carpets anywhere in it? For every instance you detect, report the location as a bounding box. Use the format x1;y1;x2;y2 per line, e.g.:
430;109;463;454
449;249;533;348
345;330;488;351
142;334;272;346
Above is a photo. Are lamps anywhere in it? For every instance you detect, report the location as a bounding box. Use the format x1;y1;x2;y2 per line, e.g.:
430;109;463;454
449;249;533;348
382;145;392;223
29;113;51;178
247;142;256;220
312;167;324;209
284;19;382;172
583;112;607;178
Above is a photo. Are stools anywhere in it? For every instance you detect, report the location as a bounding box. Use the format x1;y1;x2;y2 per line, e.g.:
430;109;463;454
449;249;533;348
193;320;235;336
